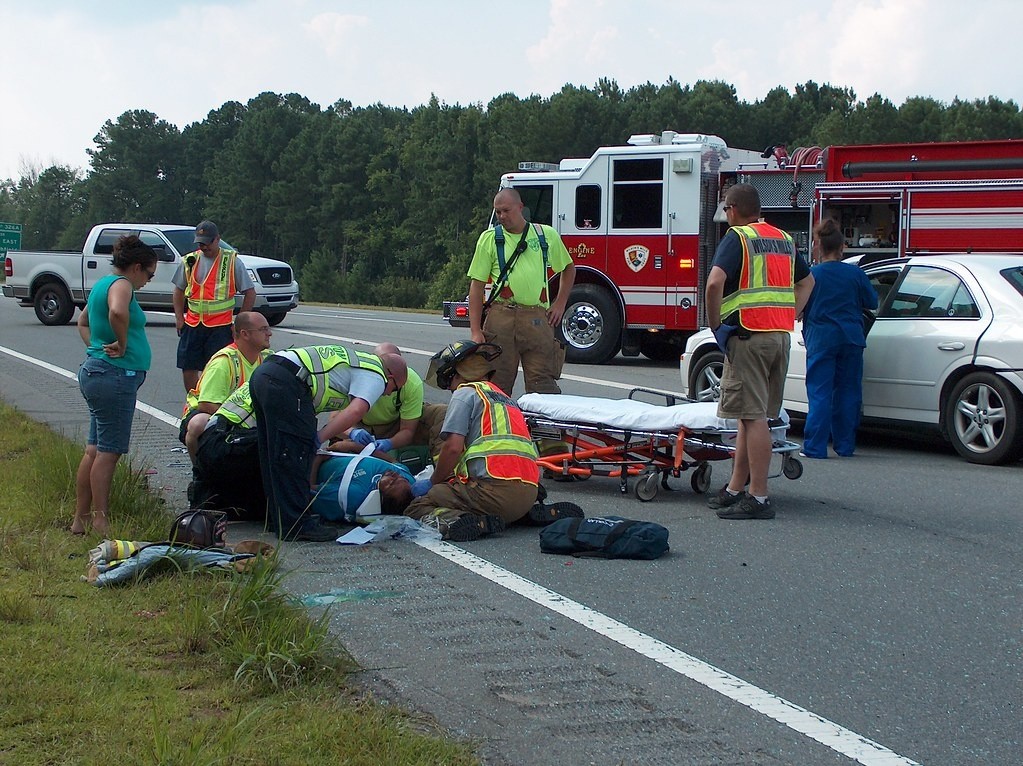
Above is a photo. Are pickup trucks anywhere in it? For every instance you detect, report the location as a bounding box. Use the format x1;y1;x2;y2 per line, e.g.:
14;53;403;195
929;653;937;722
3;222;300;326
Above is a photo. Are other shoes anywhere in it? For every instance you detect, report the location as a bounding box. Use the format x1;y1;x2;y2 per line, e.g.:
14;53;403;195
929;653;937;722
187;479;207;506
278;524;344;544
449;513;505;542
529;502;584;523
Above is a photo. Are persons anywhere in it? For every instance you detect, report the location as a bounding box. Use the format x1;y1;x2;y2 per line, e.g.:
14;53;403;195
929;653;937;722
180;312;585;542
798;218;879;458
467;188;577;483
70;236;158;535
705;183;816;518
171;221;258;392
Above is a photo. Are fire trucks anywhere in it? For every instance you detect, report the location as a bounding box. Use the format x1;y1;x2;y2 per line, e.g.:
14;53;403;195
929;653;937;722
442;130;1023;364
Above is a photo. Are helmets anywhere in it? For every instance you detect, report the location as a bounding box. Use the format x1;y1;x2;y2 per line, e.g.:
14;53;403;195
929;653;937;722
172;510;220;548
437;340;479;383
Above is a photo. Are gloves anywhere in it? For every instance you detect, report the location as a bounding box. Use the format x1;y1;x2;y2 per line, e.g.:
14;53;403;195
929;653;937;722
374;438;391;451
713;324;738;353
348;429;376;446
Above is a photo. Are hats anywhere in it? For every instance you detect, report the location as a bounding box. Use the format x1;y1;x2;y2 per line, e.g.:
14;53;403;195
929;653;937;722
193;221;218;245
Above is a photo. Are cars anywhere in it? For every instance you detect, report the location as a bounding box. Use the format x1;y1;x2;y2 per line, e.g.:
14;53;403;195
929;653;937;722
678;253;1023;465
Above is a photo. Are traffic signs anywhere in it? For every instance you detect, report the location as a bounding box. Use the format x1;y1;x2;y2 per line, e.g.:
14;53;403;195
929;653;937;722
0;222;22;263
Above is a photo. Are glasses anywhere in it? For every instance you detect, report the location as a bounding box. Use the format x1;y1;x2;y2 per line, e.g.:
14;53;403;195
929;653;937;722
245;326;271;333
723;203;737;212
141;263;155;281
387;368;399;393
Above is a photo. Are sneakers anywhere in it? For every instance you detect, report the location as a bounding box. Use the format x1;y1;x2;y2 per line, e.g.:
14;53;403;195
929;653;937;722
707;484;746;509
716;495;777;520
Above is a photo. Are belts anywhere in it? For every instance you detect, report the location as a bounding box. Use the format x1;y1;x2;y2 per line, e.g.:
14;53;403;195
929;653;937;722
267;355;300;377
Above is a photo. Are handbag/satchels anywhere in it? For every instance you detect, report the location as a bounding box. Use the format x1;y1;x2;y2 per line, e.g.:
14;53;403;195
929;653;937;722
539;516;672;561
